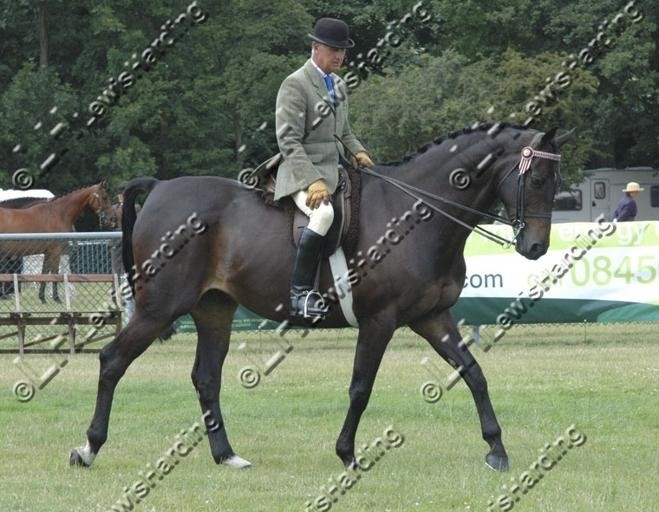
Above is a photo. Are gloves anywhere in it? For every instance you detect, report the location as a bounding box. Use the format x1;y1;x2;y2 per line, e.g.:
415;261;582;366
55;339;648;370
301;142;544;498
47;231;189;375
353;151;374;170
306;180;333;210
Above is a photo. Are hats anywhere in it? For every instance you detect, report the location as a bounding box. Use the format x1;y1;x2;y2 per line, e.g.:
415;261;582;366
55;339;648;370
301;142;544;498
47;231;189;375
307;16;357;50
621;180;645;194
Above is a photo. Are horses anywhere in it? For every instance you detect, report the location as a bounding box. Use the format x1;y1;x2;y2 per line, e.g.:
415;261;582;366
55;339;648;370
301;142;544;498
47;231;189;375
0;175;119;307
69;120;579;476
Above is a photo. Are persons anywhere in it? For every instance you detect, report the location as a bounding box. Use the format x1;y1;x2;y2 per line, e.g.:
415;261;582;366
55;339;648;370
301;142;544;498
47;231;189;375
613;181;645;221
273;17;375;318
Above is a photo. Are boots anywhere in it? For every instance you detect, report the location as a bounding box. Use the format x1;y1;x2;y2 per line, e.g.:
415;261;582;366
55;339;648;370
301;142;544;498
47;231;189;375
289;227;329;314
